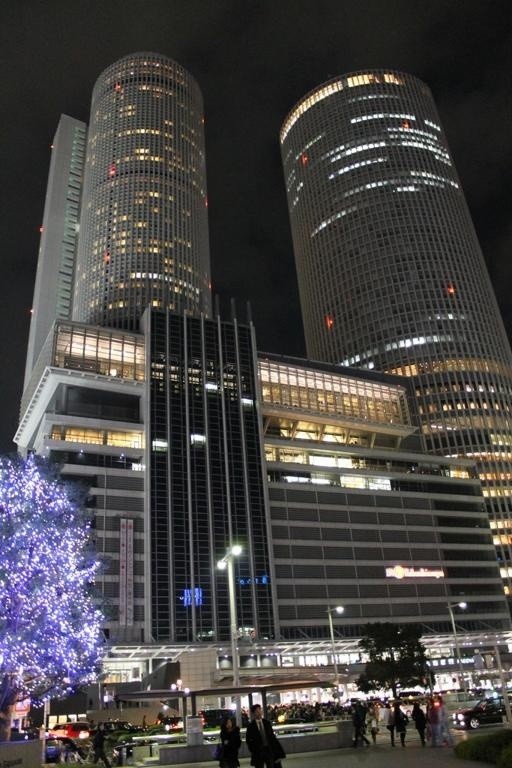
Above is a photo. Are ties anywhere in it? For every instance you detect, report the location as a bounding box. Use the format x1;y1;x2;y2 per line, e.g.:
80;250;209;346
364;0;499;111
259;722;266;745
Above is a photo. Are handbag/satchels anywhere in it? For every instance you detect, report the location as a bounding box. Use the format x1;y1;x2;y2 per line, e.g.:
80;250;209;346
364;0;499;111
212;744;221;760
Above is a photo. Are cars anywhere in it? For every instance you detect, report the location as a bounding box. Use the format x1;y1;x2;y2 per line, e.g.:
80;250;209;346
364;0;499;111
340;688;499;712
9;716;184;765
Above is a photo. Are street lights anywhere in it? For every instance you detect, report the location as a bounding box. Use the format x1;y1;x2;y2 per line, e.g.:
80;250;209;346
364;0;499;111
171;680;190;717
446;601;471;692
217;544;244;729
326;605;344;704
104;694;109;709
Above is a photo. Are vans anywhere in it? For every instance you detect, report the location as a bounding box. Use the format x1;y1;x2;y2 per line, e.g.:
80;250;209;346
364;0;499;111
196;707;249;731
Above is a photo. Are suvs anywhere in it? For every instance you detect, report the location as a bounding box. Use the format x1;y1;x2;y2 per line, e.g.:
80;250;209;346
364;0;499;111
452;695;511;731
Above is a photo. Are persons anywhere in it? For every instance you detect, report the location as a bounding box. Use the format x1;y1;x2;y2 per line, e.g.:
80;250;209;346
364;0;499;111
246;704;286;767
92;722;110;766
268;702;345;734
218;716;242;766
351;695;452;747
